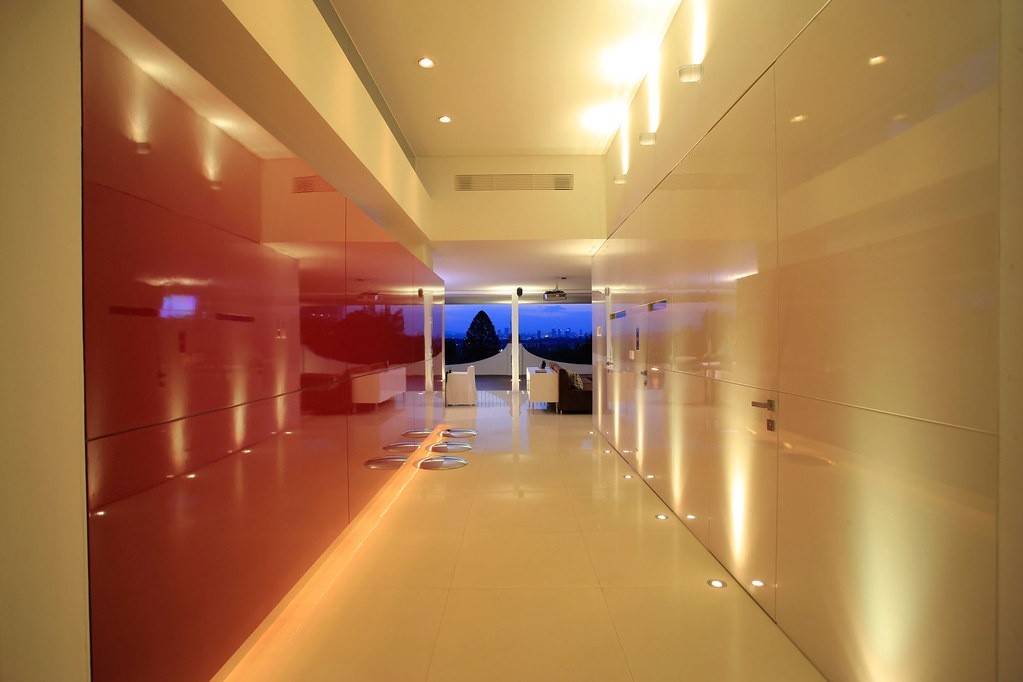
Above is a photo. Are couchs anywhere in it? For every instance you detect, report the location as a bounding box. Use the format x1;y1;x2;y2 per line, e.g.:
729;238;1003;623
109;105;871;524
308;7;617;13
550;361;593;415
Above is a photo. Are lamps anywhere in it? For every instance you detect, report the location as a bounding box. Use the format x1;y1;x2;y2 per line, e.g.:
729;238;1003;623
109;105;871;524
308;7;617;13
543;291;567;301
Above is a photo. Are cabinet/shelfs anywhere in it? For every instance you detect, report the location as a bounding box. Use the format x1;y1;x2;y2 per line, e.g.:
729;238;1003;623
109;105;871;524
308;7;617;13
526;367;559;413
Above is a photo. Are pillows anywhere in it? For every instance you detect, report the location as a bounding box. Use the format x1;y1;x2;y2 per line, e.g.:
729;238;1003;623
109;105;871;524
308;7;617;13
566;368;585;390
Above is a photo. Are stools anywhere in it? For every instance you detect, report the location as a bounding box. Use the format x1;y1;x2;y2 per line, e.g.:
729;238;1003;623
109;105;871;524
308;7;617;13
446;366;477;406
